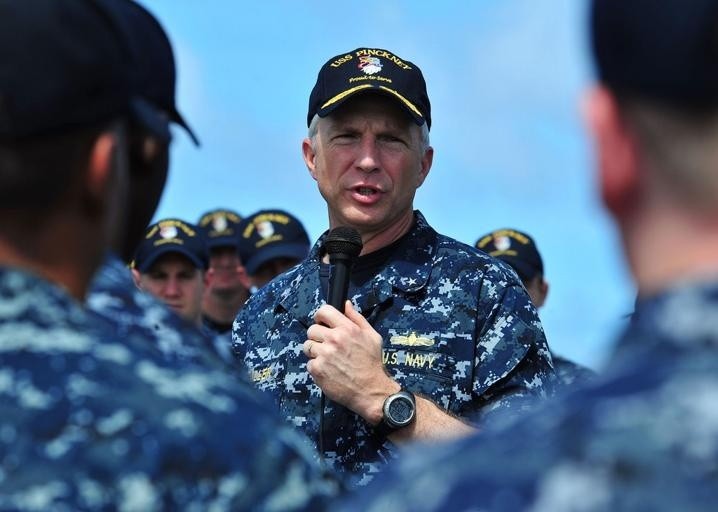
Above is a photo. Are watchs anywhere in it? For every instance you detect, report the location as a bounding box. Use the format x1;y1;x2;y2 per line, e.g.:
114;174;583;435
374;387;416;443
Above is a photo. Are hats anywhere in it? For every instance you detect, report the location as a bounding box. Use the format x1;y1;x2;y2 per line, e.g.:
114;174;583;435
237;210;310;275
198;210;241;245
134;219;209;275
0;0;199;146
476;229;542;277
308;49;432;130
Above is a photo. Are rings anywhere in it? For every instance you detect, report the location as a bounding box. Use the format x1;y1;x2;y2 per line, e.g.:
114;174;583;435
308;342;315;357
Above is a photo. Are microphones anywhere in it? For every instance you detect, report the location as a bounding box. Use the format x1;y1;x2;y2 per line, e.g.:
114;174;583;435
325;226;363;327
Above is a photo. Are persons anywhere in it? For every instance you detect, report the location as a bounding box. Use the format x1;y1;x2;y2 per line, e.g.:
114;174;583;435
196;208;250;338
472;228;596;402
236;209;311;293
1;1;359;511
135;217;218;340
318;1;716;512
231;47;561;490
